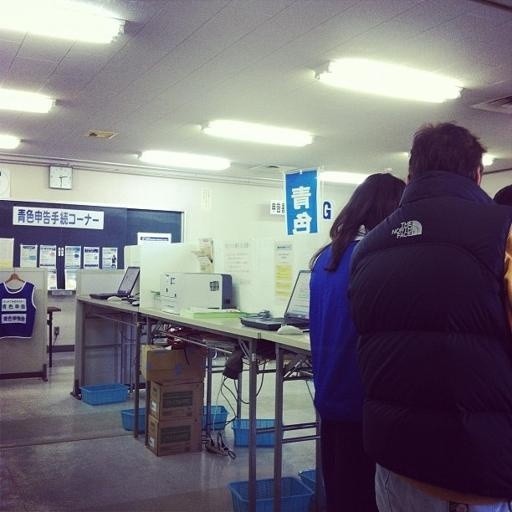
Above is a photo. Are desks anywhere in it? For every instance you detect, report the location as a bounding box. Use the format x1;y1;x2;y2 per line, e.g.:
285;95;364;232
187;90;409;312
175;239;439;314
134;302;310;511
46;306;61;367
258;326;319;511
75;289;239;441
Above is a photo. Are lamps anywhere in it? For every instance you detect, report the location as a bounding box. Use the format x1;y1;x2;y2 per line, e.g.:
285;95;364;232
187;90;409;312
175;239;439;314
313;62;463;104
0;3;134;44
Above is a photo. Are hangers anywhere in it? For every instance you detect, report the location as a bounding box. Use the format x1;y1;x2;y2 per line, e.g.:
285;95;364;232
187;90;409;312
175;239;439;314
4;267;26;286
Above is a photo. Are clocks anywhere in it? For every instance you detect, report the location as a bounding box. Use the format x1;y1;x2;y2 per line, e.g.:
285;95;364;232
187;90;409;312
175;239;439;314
49;165;74;191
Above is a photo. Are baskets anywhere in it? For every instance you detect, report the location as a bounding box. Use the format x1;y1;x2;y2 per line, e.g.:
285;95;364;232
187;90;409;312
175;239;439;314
203;405;228;430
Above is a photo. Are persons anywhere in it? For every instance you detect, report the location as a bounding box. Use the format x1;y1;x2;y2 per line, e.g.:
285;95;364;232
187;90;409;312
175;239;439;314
346;121;511;512
309;173;404;511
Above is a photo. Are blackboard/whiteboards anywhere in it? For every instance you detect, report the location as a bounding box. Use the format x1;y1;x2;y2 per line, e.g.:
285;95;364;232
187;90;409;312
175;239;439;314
0;198;184;295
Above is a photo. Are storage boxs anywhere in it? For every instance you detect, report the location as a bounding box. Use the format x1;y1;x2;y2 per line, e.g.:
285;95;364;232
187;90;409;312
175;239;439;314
201;404;230;431
149;378;205;423
147;413;203;456
139;343;206;384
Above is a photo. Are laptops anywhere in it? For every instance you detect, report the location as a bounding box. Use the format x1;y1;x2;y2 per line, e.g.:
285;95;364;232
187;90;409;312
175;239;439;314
241;270;313;331
89;267;140;299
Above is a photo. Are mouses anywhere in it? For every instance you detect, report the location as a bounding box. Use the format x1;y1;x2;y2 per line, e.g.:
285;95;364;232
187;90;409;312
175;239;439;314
107;296;121;300
277;325;303;335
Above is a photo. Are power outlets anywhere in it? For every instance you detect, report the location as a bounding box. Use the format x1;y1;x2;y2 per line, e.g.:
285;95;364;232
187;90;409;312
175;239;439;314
54;326;59;335
